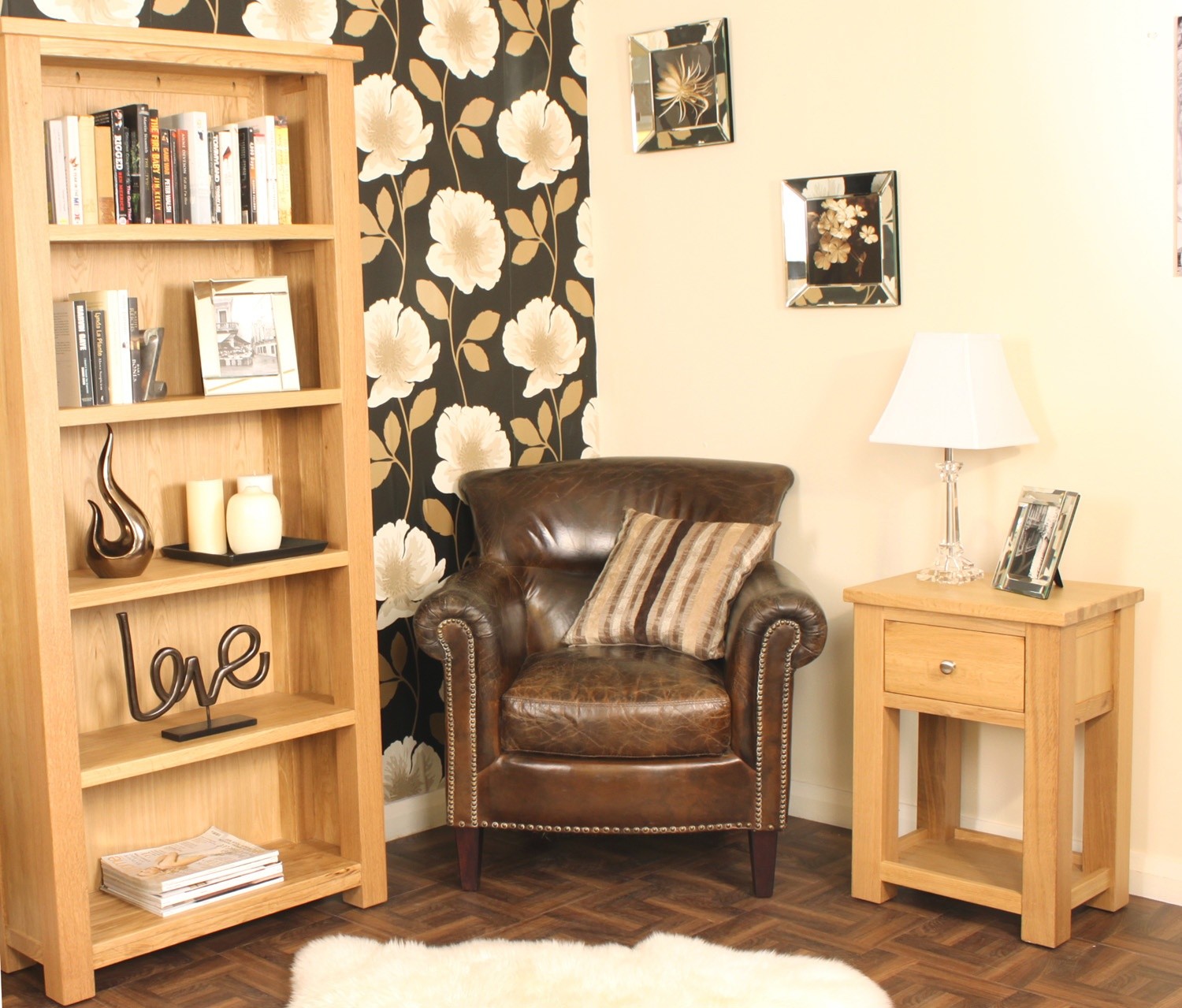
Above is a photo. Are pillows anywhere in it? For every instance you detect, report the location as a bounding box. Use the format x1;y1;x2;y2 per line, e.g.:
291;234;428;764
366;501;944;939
559;508;781;661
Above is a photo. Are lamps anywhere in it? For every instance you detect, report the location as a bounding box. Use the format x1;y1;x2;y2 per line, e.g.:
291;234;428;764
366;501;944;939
870;330;1039;584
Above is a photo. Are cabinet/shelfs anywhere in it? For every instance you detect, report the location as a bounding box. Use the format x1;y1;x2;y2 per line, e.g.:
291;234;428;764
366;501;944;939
843;564;1142;952
0;14;389;1006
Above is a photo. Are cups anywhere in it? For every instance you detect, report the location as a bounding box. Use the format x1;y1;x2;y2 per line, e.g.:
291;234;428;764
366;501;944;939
186;472;274;555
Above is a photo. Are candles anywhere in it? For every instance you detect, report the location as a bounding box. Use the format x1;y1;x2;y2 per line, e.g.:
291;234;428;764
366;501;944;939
185;478;227;554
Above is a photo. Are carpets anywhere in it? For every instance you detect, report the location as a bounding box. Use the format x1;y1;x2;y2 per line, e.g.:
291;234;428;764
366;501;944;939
287;935;900;1007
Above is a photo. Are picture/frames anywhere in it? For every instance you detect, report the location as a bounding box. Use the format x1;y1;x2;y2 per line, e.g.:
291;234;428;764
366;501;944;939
187;274;302;398
994;489;1082;596
626;17;730;153
782;170;902;308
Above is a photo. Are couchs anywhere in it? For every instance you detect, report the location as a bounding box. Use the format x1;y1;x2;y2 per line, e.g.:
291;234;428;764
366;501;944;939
411;458;828;897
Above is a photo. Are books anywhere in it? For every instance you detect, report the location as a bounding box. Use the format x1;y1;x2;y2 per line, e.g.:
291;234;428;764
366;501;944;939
44;104;291;225
52;290;142;408
99;827;285;918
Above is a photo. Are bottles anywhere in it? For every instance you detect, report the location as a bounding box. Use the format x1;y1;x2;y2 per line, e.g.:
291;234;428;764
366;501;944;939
227;486;283;556
